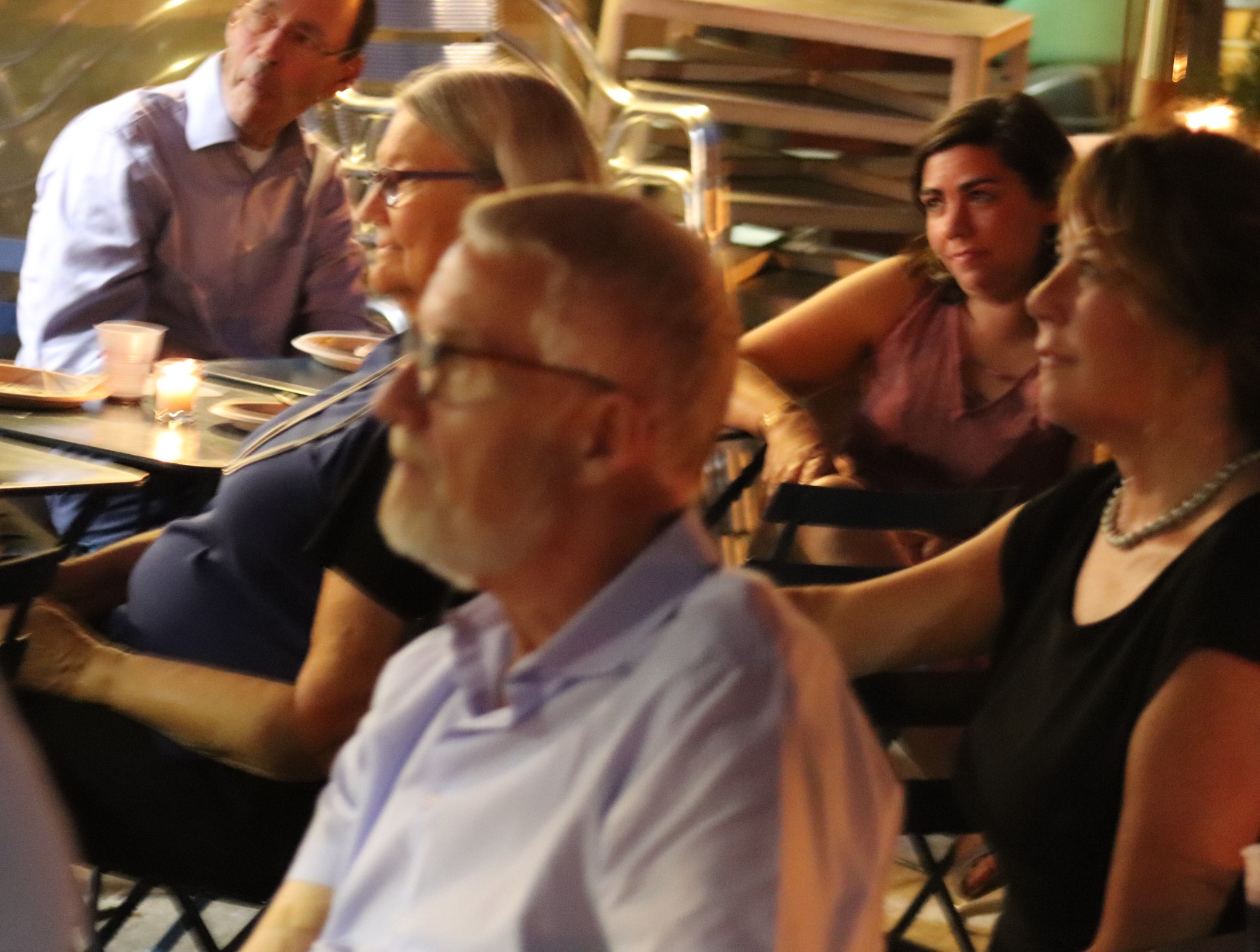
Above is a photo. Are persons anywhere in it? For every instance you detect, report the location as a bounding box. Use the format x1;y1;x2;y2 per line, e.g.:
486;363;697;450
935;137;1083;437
210;178;905;951
693;89;1122;896
740;117;1260;952
1;65;615;911
0;1;392;427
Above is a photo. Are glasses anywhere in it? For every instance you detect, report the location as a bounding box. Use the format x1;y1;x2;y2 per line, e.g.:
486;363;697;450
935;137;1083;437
238;0;358;59
337;163;497;214
389;326;629;400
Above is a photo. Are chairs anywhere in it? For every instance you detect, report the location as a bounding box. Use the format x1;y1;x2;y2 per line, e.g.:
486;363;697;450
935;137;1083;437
705;430;1025;952
318;0;731;275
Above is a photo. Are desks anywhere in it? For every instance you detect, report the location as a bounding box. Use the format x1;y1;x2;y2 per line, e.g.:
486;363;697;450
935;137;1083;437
0;355;362;671
591;0;1036;331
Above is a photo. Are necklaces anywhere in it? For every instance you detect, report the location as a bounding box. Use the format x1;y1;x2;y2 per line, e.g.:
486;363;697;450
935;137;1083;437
1102;448;1260;547
962;331;1022;389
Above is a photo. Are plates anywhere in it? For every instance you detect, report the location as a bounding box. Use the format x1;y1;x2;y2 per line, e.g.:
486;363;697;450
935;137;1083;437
0;363;113;409
290;332;386;372
208;397;302;432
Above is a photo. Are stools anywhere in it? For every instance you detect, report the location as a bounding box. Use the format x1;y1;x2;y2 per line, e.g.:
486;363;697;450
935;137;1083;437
71;853;268;952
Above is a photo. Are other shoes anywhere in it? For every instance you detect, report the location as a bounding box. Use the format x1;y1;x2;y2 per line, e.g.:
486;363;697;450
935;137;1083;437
892;830;961;873
955;843;1009;900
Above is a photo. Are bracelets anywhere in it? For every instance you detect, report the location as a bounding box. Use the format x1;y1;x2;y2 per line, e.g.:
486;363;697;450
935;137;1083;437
757;402;803;435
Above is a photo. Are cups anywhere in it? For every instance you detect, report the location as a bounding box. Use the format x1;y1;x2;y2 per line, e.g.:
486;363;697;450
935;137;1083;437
154;359;207;421
1241;843;1260;906
95;324;162;405
